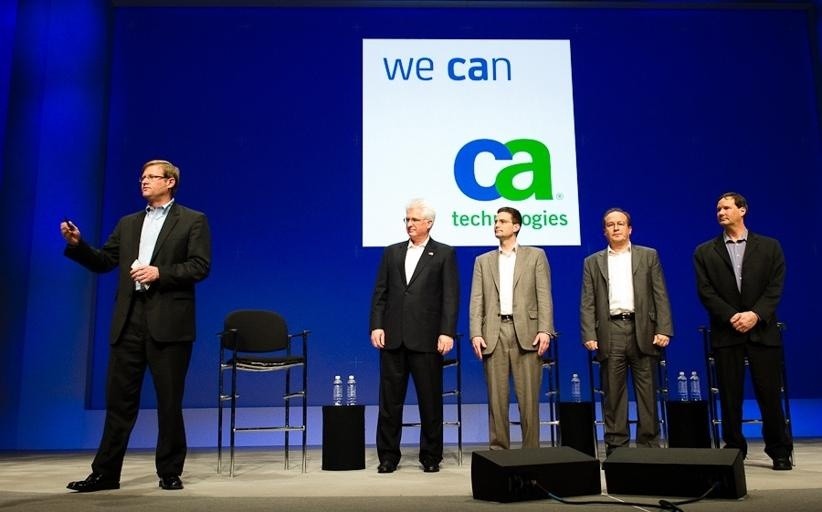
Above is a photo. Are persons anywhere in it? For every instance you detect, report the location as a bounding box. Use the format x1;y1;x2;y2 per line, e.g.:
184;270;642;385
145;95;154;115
577;206;677;455
57;159;214;492
694;190;796;469
468;206;559;455
368;199;461;473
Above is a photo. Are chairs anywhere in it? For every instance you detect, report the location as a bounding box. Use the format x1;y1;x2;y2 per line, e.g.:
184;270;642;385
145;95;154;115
402;334;464;466
589;349;668;459
697;321;794;466
509;332;560;447
216;309;311;478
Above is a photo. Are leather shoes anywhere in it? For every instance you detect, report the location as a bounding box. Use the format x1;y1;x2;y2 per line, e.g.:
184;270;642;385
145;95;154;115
158;472;183;490
771;456;792;471
67;472;121;492
377;460;398;473
423;464;439;472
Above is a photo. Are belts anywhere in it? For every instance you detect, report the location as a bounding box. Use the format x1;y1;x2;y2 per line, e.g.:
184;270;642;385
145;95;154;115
610;312;635;320
501;315;512;321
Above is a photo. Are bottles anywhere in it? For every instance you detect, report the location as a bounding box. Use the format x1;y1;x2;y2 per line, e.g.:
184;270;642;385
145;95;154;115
333;375;343;407
688;371;703;402
346;375;358;406
677;371;689;402
571;374;582;404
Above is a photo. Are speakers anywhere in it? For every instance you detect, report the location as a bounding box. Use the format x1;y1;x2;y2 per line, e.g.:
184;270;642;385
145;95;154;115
603;447;747;498
470;447;601;501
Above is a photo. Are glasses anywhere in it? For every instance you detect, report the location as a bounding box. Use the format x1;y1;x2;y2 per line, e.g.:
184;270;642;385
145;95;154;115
403;216;426;226
138;174;167;182
